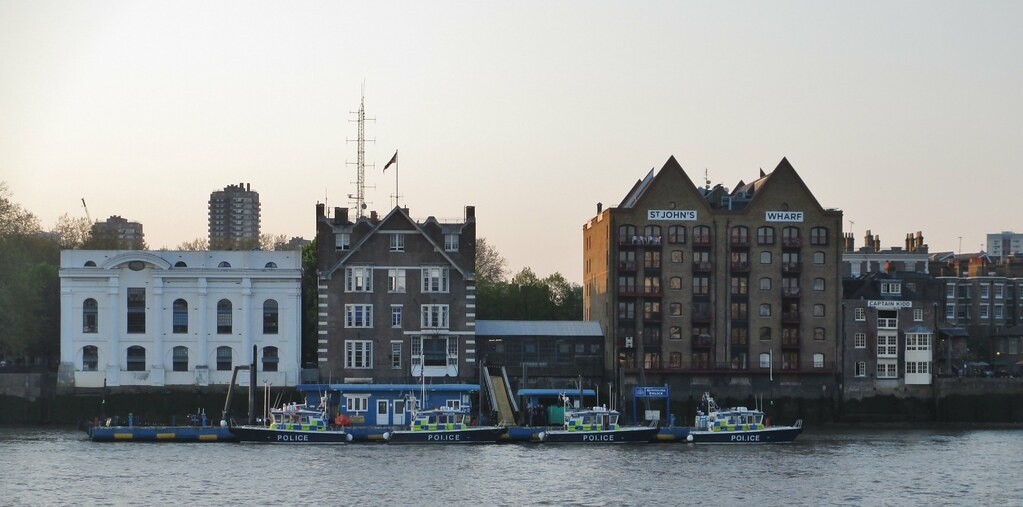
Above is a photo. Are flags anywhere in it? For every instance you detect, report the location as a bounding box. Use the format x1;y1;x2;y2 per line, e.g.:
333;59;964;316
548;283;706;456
383;151;397;172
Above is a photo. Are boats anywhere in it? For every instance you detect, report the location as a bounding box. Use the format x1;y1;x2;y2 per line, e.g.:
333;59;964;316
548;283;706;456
685;390;803;446
87;353;693;444
